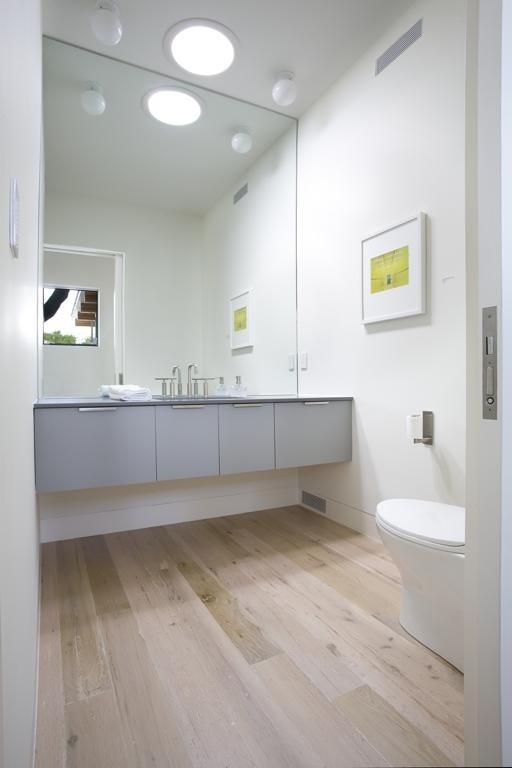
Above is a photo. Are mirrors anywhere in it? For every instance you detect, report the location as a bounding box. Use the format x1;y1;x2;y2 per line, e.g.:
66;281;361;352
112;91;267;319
42;33;299;404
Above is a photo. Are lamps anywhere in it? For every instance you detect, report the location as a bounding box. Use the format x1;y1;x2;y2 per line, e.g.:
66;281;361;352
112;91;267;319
163;19;242;77
272;67;299;109
88;1;124;48
78;80;106;116
231;126;254;154
142;86;207;127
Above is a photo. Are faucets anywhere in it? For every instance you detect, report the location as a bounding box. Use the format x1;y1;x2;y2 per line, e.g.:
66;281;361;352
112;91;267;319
171;365;182;395
187;363;198;396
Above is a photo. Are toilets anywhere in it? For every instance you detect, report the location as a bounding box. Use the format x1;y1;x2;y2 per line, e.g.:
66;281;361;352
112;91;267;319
376;497;468;676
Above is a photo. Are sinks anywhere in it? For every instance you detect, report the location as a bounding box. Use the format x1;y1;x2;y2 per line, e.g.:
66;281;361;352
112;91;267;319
153;394;245;401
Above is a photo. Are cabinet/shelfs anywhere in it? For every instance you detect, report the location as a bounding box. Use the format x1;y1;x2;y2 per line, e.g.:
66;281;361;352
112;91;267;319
221;404;276;476
156;405;220;482
275;401;351;469
34;405;156;493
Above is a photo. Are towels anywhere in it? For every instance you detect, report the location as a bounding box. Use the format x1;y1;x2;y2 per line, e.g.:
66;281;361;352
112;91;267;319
110;384;152;402
98;384;109;397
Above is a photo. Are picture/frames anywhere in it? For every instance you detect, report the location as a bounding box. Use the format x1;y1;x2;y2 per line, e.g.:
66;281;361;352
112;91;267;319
358;211;428;327
228;288;254;350
